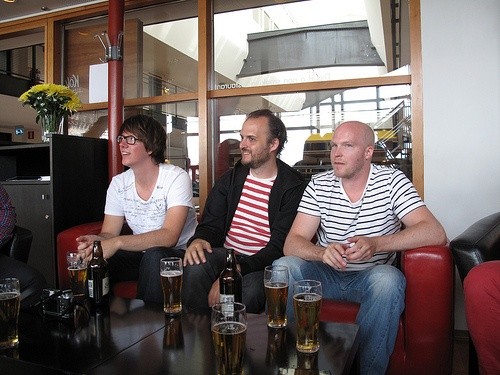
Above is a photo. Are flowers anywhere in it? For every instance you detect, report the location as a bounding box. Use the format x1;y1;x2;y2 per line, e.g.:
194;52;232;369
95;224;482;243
18;83;81;132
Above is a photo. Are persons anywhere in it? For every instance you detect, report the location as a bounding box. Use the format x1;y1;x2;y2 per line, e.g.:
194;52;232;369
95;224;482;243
269;121;447;375
0;185;49;311
179;109;311;312
213;133;335;191
75;115;198;308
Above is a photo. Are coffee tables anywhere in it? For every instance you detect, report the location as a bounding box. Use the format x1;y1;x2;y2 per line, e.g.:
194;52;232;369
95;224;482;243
0;292;361;374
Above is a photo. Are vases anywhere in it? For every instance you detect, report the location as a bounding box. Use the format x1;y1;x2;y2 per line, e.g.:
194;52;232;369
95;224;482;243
40;115;63;142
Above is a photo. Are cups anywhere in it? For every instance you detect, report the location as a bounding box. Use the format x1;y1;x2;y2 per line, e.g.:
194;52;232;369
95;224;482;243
0;277;22;349
160;257;183;314
211;302;247;375
293;279;322;353
264;265;290;329
67;249;88;300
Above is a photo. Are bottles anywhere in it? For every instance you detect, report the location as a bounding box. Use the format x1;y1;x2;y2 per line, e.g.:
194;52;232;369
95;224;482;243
87;240;109;307
219;248;242;322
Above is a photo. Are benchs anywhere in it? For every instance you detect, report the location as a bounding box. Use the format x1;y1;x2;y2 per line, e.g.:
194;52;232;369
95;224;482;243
57;220;454;374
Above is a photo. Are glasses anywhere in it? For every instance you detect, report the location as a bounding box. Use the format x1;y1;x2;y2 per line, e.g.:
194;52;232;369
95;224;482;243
116;133;144;146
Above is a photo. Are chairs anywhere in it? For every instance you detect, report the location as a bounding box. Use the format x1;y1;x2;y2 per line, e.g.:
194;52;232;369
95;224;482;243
450;212;500;375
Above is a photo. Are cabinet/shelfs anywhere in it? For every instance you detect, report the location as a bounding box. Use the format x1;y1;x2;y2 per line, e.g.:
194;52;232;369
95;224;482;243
0;132;109;290
